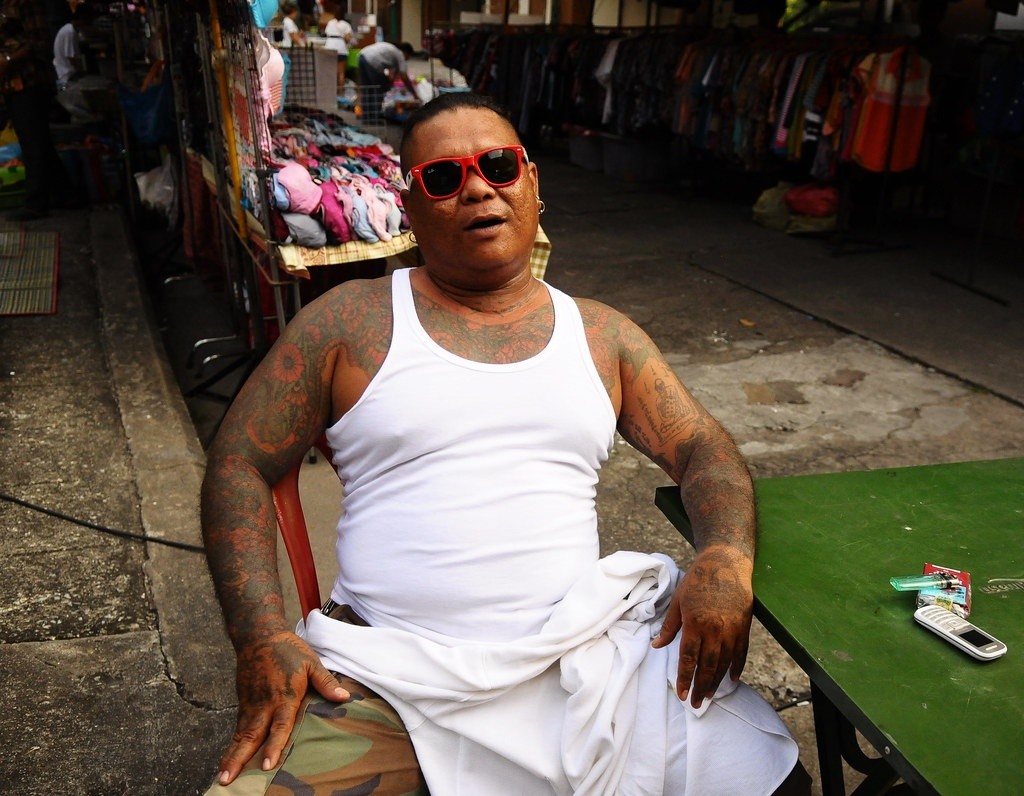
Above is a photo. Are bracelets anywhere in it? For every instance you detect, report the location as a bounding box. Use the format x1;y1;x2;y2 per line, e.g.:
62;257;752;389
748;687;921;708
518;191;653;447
6;53;15;66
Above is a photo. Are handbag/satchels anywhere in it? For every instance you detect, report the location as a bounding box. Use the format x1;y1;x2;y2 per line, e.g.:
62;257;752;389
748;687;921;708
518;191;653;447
134;153;174;210
276;40;338;108
2;51;25;94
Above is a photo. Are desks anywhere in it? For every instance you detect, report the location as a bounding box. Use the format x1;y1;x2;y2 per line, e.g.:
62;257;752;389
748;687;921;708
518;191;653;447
186;151;551;320
654;457;1024;796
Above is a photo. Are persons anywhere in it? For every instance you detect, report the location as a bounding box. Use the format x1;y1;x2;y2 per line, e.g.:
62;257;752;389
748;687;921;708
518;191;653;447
280;0;415;115
199;88;798;796
0;0;97;194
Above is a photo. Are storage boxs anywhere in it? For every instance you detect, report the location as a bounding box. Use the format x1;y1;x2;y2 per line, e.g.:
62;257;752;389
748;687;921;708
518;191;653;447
80;76;115;114
0;189;27;211
49;123;84;143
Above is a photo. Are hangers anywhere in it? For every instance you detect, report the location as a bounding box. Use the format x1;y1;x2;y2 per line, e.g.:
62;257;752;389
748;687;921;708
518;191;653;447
431;21;915;55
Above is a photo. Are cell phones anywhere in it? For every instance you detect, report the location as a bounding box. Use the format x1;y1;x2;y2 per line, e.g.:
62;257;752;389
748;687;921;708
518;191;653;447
913;605;1008;661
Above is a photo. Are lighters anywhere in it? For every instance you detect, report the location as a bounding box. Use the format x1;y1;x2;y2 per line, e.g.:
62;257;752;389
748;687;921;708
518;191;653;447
892;571;962;591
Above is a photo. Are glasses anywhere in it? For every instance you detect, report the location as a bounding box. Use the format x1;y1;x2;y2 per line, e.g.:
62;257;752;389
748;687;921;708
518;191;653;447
406;144;529;201
